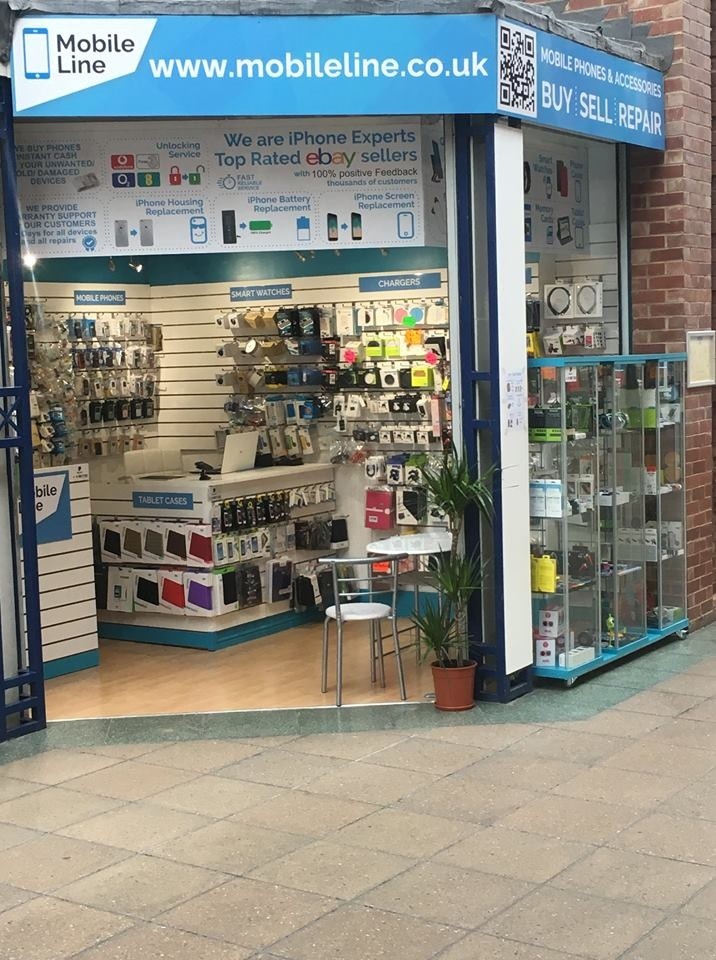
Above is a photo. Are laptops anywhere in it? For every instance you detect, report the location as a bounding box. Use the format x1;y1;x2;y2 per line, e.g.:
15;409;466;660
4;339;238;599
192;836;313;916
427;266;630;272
189;430;258;475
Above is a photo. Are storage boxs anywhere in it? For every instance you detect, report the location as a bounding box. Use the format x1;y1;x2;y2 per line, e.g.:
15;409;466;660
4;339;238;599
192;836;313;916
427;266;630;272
526;376;682;671
215;301;448;549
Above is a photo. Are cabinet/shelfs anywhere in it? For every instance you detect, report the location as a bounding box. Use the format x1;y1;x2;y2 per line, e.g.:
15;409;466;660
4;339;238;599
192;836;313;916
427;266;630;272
525;352;689;687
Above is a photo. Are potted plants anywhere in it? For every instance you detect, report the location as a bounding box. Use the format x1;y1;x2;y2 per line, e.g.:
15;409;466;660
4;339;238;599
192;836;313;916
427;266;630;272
407;447;503;710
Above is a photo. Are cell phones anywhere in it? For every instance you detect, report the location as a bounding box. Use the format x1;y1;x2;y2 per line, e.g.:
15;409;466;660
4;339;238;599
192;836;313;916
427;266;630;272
114;219;129;247
327;213;338;241
351;212;363;239
139;219;154;246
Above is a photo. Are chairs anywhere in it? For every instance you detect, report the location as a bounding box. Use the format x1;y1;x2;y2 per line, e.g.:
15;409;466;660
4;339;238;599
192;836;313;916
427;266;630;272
318;552;411;706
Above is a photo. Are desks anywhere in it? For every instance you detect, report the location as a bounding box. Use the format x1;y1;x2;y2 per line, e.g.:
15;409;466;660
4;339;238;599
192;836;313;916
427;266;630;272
366;531;452;664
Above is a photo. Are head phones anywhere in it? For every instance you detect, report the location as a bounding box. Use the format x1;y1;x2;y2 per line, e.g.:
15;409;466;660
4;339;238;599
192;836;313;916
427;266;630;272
576;285;597;315
548;286;571;315
